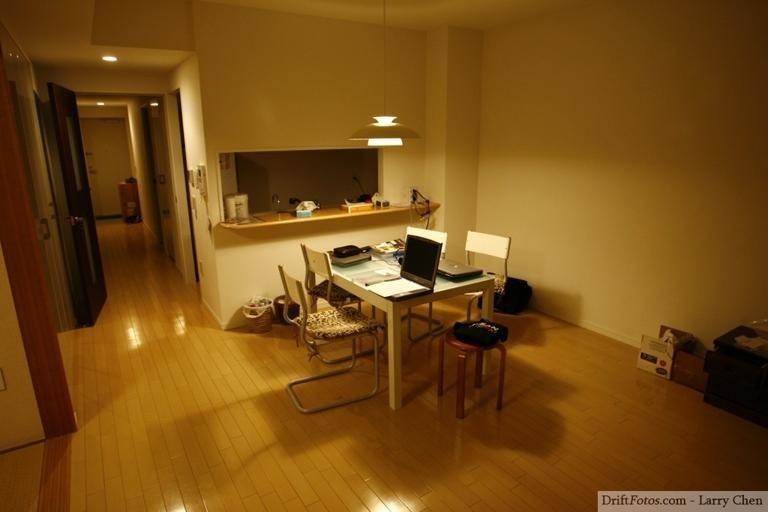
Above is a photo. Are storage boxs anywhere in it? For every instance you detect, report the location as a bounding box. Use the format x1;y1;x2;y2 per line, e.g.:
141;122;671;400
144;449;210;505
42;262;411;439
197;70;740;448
637;324;767;428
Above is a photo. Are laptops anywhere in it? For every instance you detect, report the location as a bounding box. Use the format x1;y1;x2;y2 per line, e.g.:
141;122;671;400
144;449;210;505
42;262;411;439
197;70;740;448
437;255;483;282
364;234;442;302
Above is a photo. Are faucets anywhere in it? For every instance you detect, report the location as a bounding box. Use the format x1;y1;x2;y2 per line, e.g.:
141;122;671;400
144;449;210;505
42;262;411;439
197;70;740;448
273;194;281;205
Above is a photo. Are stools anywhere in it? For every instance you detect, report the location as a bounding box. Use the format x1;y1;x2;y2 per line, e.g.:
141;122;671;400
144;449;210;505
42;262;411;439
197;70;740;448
436;327;506;419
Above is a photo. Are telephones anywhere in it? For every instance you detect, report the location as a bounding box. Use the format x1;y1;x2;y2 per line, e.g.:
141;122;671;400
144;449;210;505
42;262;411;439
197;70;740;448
194;164;207;195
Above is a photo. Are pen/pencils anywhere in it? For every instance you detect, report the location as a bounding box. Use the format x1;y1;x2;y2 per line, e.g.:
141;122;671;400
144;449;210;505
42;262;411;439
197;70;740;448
365;280;389;286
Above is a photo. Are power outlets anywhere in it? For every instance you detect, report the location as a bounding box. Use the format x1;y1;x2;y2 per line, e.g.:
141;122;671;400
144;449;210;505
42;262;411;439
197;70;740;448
408;185;421;206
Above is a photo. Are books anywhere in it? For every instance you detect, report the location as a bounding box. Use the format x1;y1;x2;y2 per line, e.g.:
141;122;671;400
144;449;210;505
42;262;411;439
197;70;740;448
326;238;407;268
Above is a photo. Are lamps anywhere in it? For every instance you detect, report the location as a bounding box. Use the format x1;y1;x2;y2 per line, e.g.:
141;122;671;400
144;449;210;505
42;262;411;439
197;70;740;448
348;0;422;147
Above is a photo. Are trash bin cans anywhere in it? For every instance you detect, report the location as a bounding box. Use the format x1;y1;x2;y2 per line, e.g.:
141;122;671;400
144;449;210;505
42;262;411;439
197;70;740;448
242;298;273;334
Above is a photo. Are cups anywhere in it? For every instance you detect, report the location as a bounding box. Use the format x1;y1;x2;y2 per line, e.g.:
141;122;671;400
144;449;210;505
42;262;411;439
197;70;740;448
224;193;249;222
